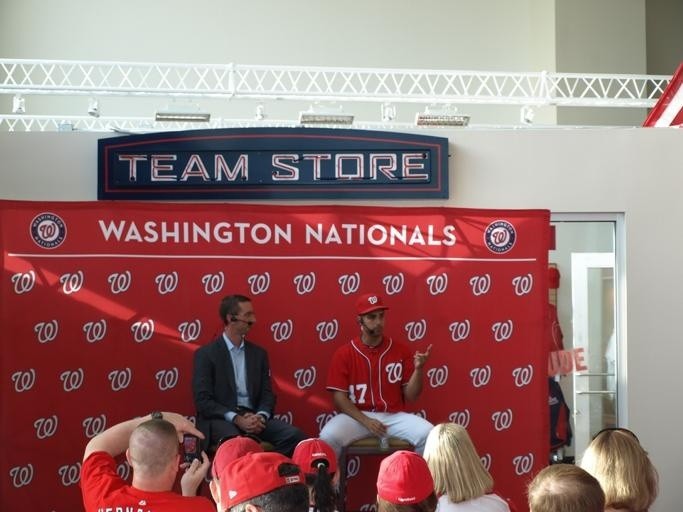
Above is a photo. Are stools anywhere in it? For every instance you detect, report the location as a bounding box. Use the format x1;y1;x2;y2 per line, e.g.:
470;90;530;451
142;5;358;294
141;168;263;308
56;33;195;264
342;435;413;511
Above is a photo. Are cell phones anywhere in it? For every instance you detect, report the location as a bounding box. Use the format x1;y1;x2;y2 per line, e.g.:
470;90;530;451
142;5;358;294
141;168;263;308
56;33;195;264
182;435;201;465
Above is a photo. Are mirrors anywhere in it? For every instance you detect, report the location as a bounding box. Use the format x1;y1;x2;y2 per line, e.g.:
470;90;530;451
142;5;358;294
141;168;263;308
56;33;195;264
547;211;631;468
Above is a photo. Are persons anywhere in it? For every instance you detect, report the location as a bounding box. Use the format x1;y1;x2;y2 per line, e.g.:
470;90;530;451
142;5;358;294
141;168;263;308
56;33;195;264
580;428;659;511
209;436;264;511
80;411;215;511
320;293;435;451
528;464;605;511
423;423;510;512
193;294;307;457
293;439;338;512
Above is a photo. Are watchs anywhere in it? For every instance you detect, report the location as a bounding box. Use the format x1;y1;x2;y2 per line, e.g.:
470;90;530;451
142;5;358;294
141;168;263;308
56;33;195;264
149;411;163;419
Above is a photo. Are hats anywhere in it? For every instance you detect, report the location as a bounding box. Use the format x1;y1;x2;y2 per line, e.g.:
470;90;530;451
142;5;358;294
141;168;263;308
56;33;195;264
353;293;389;316
291;437;336;475
211;435;265;485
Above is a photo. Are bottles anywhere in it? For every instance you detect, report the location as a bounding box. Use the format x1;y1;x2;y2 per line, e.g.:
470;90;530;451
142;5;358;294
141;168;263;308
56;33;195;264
379;423;389;450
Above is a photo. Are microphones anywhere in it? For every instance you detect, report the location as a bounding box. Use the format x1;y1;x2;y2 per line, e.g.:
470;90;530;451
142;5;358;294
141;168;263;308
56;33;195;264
362;323;375;336
234;318;253;327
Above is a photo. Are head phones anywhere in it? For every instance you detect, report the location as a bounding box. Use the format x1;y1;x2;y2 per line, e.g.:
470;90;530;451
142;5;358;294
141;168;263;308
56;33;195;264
230;294;240;323
356;315;363;324
